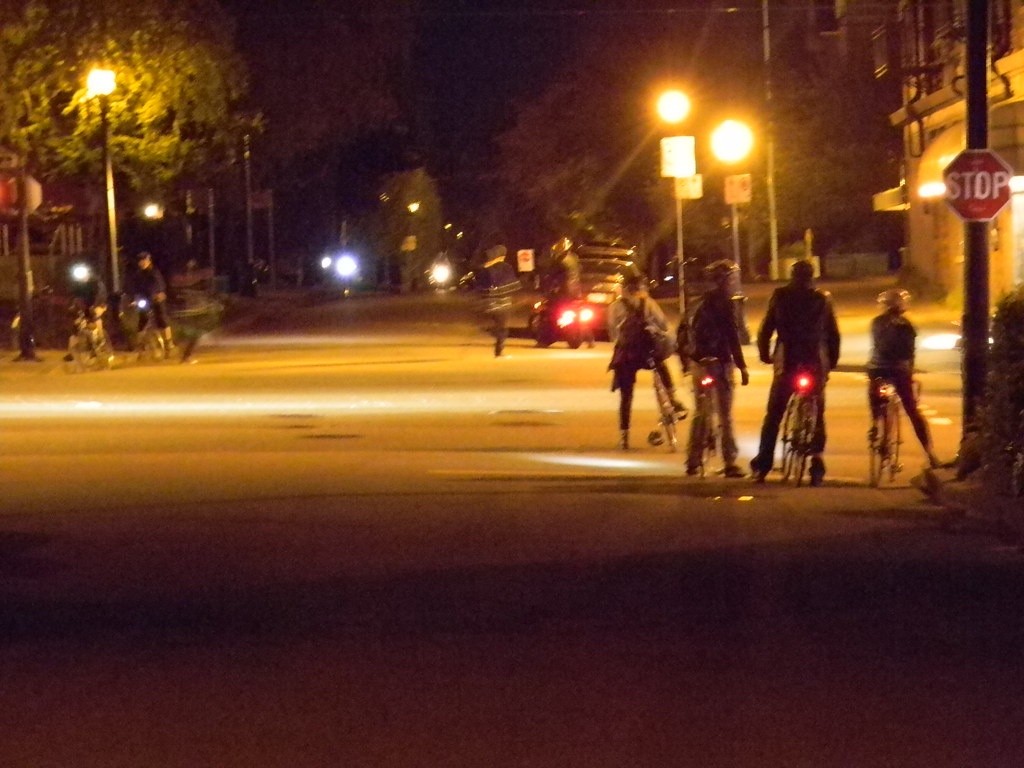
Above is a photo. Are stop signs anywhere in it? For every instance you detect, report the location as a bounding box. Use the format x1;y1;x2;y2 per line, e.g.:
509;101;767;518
942;149;1014;222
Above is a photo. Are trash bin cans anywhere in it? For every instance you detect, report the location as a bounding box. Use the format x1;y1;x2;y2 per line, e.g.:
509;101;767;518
778;255;822;282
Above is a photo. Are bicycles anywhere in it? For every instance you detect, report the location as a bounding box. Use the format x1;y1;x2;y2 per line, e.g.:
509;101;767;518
683;357;722;478
869;368;925;487
781;366;821;487
65;305;114;372
126;295;173;364
643;347;686;450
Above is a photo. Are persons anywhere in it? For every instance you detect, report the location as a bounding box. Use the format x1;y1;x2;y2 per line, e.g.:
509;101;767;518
675;258;745;477
476;244;524;356
134;250;176;360
63;254;114;370
748;259;843;484
532;234;597;350
608;278;686;449
863;288;946;468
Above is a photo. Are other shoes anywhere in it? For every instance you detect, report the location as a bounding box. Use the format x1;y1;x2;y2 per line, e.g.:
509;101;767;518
723;465;747;478
164;338;175;350
686;465;697;474
811;473;823;484
750;470;764;481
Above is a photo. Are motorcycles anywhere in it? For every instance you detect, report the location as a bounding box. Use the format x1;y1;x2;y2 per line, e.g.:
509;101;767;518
526;276;588;351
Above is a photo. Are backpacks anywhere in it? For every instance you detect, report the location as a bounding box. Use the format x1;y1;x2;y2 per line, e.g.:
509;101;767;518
620;296;658;360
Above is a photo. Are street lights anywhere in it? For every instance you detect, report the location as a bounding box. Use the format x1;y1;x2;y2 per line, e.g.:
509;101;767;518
720;121;751;345
403;202;418;295
661;88;696;354
92;69;124;338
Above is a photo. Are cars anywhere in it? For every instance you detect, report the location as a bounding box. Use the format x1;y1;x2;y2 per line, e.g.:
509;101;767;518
529;258;648;341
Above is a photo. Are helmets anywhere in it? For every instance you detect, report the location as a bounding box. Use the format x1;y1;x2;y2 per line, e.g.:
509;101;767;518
880;289;911;311
706;260;740;279
